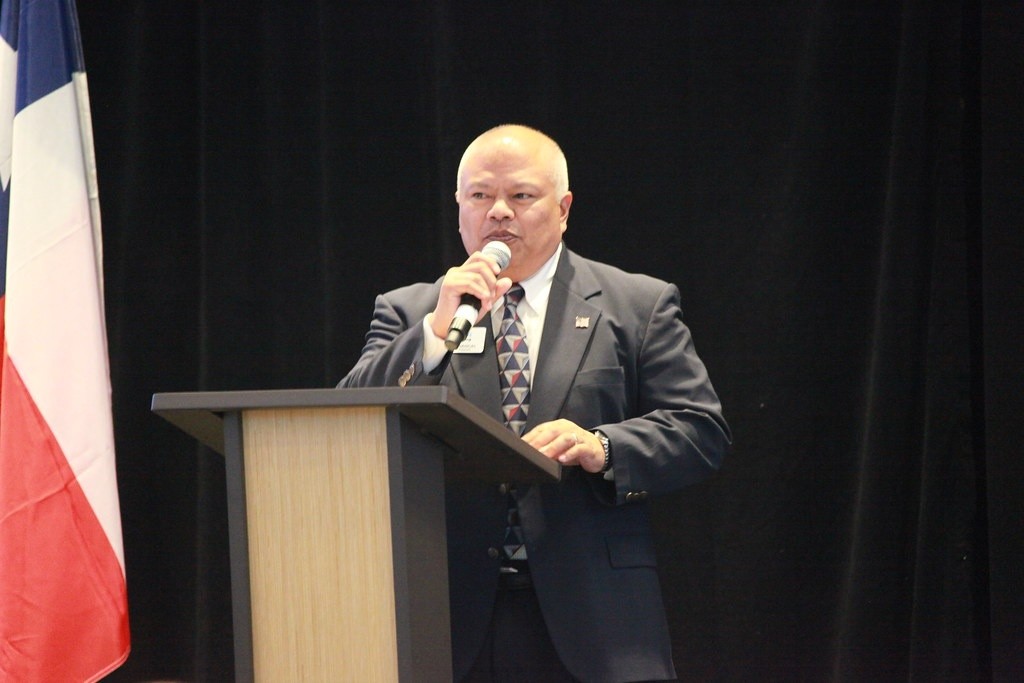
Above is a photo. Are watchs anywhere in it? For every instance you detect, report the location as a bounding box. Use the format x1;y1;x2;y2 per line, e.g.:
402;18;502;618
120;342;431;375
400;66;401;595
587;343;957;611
595;431;612;472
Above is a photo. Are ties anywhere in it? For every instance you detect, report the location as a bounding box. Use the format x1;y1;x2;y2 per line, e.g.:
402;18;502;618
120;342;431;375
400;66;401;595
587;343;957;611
491;281;536;574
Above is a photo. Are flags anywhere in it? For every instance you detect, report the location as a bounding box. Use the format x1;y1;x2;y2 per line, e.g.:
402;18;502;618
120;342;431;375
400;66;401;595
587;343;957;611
0;0;131;683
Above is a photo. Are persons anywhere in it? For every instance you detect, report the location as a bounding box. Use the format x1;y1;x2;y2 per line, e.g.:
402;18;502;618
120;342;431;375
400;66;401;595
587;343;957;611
335;125;733;683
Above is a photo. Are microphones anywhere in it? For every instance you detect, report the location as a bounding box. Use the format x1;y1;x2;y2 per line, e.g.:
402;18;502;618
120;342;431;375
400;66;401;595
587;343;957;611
445;240;511;351
